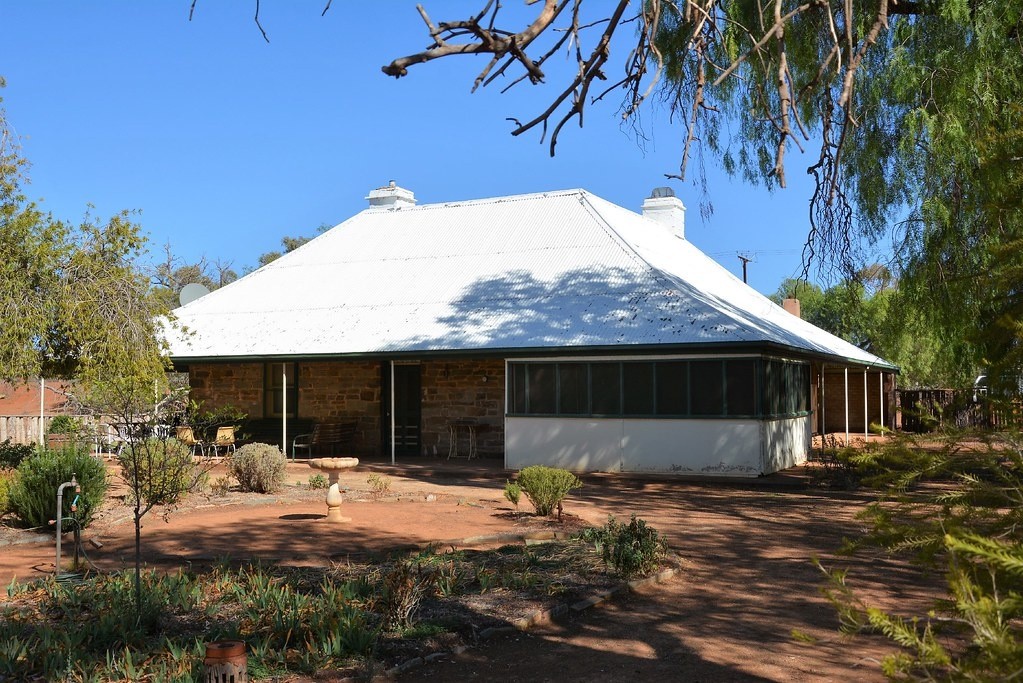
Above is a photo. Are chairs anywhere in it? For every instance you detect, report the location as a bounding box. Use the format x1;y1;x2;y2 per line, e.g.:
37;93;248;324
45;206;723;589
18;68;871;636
207;426;235;462
177;426;204;458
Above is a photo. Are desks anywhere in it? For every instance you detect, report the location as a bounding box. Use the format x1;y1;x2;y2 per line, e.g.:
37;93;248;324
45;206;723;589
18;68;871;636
110;422;145;454
445;416;480;461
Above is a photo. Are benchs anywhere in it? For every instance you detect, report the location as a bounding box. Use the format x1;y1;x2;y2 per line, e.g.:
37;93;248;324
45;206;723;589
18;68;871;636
292;420;359;463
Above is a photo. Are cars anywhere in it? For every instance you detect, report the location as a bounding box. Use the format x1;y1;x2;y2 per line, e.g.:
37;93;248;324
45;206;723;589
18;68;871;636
970;375;1010;405
